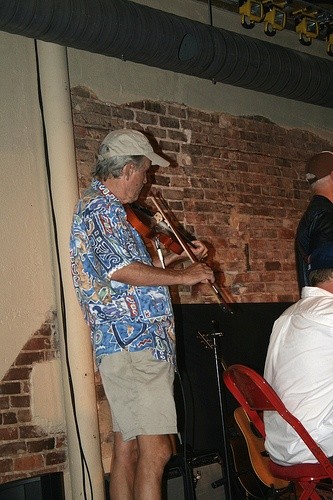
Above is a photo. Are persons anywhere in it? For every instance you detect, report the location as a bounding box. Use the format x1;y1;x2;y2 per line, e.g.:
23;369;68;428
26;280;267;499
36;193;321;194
70;128;215;500
294;150;333;299
260;243;333;466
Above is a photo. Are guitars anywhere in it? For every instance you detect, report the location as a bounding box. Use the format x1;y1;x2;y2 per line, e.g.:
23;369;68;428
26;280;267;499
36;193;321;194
195;329;294;498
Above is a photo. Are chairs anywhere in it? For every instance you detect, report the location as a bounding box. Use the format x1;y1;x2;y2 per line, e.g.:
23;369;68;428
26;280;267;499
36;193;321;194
222;365;333;500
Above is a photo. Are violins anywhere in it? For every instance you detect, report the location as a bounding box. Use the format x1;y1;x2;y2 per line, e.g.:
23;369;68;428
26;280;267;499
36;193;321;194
123;201;208;264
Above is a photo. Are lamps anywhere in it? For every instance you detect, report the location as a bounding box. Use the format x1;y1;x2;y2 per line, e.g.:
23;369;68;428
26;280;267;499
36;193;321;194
238;0;333;58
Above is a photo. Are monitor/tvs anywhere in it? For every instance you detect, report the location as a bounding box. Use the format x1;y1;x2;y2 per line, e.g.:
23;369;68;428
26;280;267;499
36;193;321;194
162;301;297;481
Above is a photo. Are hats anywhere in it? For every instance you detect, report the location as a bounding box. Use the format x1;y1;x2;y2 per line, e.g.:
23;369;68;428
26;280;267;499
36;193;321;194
94;128;170;168
304;152;333;184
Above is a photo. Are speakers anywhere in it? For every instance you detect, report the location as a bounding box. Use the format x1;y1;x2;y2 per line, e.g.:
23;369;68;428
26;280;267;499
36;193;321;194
0;471;65;500
